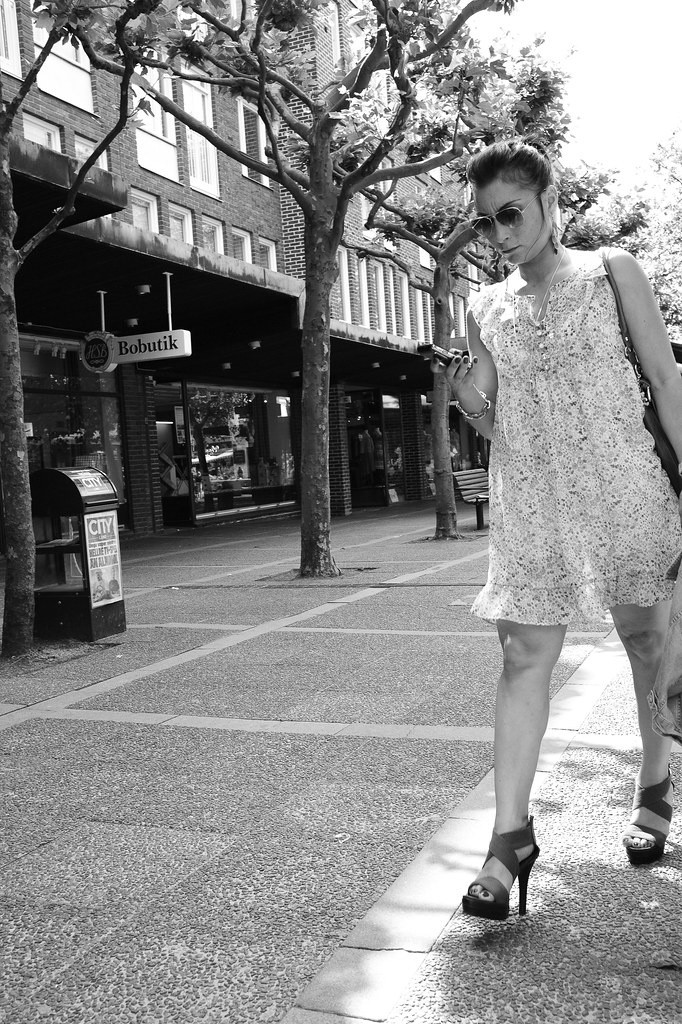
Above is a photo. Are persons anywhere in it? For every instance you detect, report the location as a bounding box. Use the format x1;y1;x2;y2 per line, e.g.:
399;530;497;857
371;427;384;452
428;133;682;920
360;429;374;485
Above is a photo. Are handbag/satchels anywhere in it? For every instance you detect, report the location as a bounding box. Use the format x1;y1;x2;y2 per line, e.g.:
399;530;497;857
601;245;682;498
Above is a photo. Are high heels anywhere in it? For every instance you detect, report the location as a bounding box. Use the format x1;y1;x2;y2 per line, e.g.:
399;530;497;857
626;768;675;865
462;815;540;920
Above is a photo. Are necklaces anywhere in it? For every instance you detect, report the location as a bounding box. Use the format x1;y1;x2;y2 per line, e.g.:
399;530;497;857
512;246;568;391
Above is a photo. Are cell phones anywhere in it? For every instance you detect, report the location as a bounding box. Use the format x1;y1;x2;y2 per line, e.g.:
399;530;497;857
418;344;472;375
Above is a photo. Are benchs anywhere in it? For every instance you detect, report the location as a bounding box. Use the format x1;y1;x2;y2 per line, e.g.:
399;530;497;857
451;468;490;530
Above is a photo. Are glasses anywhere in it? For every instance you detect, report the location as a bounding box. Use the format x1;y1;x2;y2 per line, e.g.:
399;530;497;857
470;193;545;240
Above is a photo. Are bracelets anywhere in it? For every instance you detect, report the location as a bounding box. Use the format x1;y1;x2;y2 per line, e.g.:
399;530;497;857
456;389;491;420
